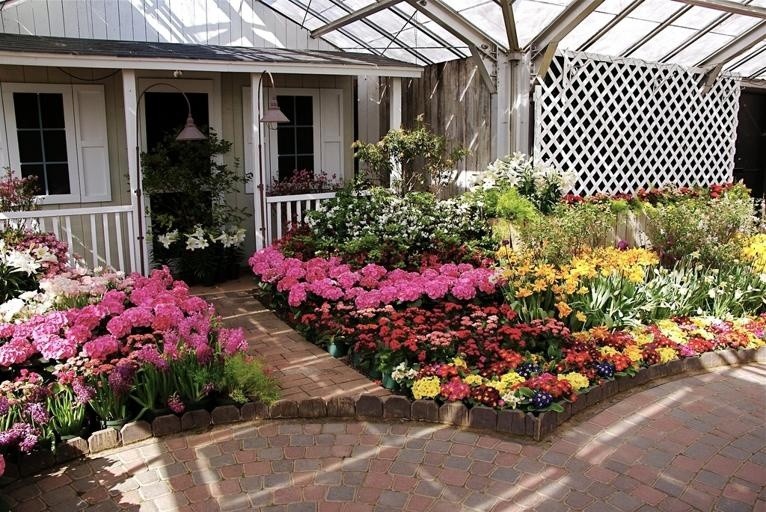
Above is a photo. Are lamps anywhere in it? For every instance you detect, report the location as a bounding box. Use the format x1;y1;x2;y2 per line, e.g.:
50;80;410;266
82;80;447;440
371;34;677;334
253;69;292;248
132;78;208;274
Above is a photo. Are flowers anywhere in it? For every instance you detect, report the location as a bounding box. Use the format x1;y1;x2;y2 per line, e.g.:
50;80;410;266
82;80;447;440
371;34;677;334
2;174;250;460
259;147;765;414
